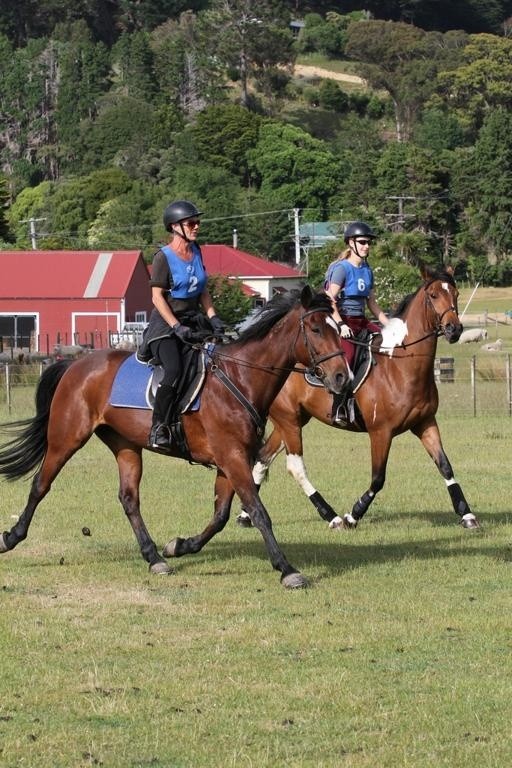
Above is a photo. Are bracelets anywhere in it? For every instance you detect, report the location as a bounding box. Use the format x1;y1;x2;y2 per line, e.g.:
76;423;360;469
337;321;343;326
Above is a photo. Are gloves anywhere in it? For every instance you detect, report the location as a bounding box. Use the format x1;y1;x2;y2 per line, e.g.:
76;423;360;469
174;323;192;341
210;315;226;337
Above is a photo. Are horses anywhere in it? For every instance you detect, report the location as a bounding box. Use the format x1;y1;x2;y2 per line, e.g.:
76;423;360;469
0;285;354;588
236;260;480;529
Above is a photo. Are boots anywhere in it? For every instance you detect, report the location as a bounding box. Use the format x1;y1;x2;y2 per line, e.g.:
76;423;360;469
330;394;346;428
147;382;176;449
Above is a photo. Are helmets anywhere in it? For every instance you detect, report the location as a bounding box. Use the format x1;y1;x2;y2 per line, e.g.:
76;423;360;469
344;222;378;245
163;200;204;232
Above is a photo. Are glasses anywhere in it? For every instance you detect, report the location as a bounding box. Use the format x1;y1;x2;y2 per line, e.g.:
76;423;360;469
352;239;372;246
182;219;200;228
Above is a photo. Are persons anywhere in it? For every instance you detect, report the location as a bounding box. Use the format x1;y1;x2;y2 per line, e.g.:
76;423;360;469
145;200;224;450
324;222;390;425
